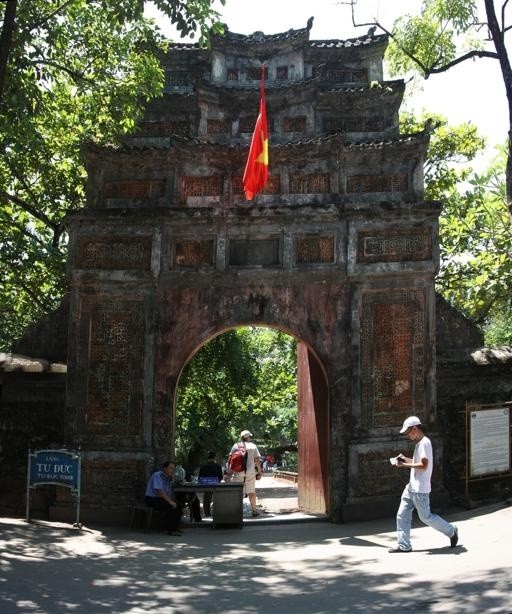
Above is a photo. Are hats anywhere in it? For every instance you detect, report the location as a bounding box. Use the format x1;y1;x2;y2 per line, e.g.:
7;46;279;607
241;430;253;438
399;416;421;434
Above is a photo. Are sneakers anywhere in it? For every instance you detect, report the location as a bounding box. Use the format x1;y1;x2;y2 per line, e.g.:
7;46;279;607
252;510;259;516
450;527;458;548
388;547;412;552
170;529;184;536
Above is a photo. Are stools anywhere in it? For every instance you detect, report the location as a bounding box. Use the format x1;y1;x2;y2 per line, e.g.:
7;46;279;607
130;504;153;534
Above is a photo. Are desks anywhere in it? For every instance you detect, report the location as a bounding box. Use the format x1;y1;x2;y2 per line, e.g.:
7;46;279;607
173;483;244;529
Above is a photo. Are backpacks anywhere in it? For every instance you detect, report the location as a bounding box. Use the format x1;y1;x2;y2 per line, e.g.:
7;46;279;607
229;442;247;472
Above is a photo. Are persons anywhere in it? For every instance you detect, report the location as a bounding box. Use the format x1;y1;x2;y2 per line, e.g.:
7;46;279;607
260;454;287;473
199;451;224;516
228;430;262;517
144;462;184;536
386;416;459;553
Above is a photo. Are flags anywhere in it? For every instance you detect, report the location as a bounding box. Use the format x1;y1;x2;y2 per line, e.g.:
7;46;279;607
242;101;270;201
